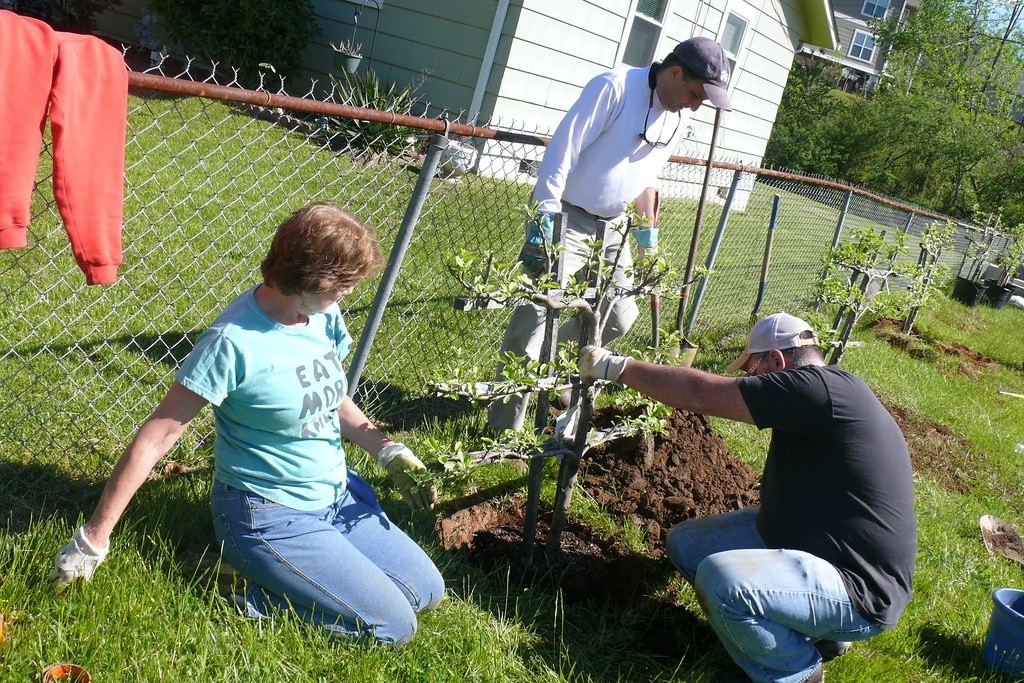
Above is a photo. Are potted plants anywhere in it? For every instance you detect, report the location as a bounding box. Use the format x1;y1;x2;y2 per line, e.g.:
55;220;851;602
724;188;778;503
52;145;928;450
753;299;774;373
951;210;1005;309
989;224;1024;305
978;222;1024;309
148;50;167;70
330;40;364;75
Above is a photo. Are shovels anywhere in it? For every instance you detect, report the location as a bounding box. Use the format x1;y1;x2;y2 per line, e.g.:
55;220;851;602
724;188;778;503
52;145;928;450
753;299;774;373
658;109;724;370
979;513;1024;569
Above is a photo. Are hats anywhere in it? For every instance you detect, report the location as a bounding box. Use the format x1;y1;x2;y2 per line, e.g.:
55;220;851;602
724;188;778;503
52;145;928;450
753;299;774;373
725;311;820;372
674;37;733;112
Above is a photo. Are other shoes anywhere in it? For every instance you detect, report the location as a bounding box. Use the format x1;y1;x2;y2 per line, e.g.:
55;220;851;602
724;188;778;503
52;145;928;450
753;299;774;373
187;563;251;600
805;661;824;683
817;637;853;659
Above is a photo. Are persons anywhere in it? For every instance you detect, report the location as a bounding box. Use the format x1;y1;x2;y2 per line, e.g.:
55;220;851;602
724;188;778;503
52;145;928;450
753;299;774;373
482;37;734;457
49;202;446;645
576;308;918;683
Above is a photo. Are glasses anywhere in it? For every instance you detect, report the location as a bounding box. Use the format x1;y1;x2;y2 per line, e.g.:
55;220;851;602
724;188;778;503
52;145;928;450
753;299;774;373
640;103;681;149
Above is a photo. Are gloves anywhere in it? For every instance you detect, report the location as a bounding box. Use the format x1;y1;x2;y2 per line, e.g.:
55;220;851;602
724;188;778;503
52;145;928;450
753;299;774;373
632;228;659;273
49;526;110;595
578;346;633;388
376;442;437;513
519;214;556;277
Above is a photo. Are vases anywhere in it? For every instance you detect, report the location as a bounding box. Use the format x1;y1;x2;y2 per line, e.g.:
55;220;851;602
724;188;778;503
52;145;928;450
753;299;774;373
441;140;478;184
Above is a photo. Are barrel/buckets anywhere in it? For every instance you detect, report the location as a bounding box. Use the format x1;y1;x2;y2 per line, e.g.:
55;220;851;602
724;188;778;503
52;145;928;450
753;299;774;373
984;587;1024;679
